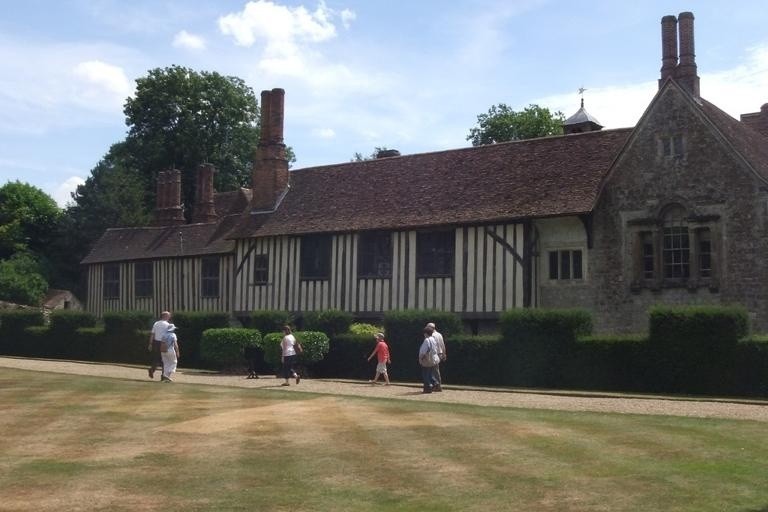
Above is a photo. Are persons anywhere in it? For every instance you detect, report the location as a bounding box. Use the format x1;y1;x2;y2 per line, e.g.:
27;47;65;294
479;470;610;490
279;326;302;386
418;326;439;393
146;311;172;380
159;323;179;382
367;333;392;386
245;335;259;379
427;322;447;392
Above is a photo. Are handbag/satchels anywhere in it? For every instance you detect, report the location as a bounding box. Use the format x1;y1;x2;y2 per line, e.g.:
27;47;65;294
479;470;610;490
294;342;303;352
422;350;434;362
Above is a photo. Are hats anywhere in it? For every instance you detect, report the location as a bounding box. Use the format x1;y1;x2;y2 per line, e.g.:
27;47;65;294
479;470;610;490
375;333;385;340
166;324;176;331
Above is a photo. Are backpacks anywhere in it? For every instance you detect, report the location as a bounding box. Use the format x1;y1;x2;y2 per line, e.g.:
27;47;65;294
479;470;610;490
160;333;174;352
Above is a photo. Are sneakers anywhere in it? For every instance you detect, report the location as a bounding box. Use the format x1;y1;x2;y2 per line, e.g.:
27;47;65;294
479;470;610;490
148;369;153;377
282;383;290;386
369;380;376;384
162;375;171;381
296;375;300;383
382;383;390;386
431;387;442;391
422;390;432;393
